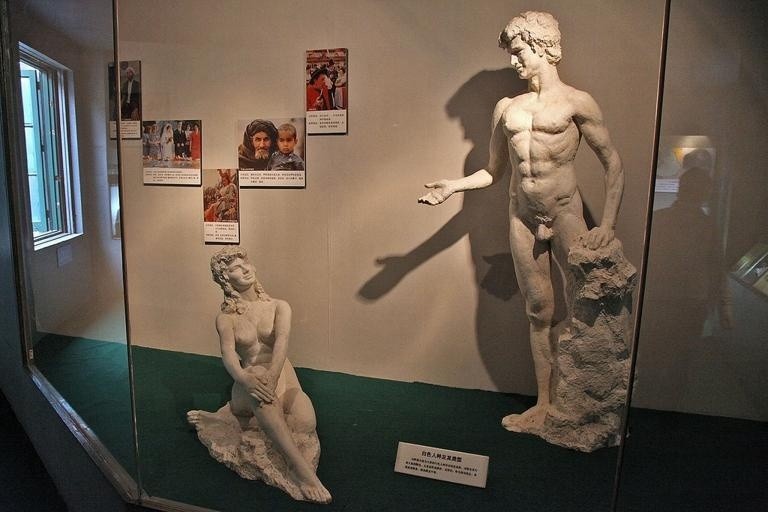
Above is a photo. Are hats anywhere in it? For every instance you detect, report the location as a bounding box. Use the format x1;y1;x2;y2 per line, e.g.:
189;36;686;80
309;68;327;84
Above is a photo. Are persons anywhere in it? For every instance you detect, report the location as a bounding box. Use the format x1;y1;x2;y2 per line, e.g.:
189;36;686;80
120;67;140;121
213;172;237;222
306;51;346;110
143;121;201;161
239;120;278;171
415;9;626;437
209;244;334;503
266;124;305;171
